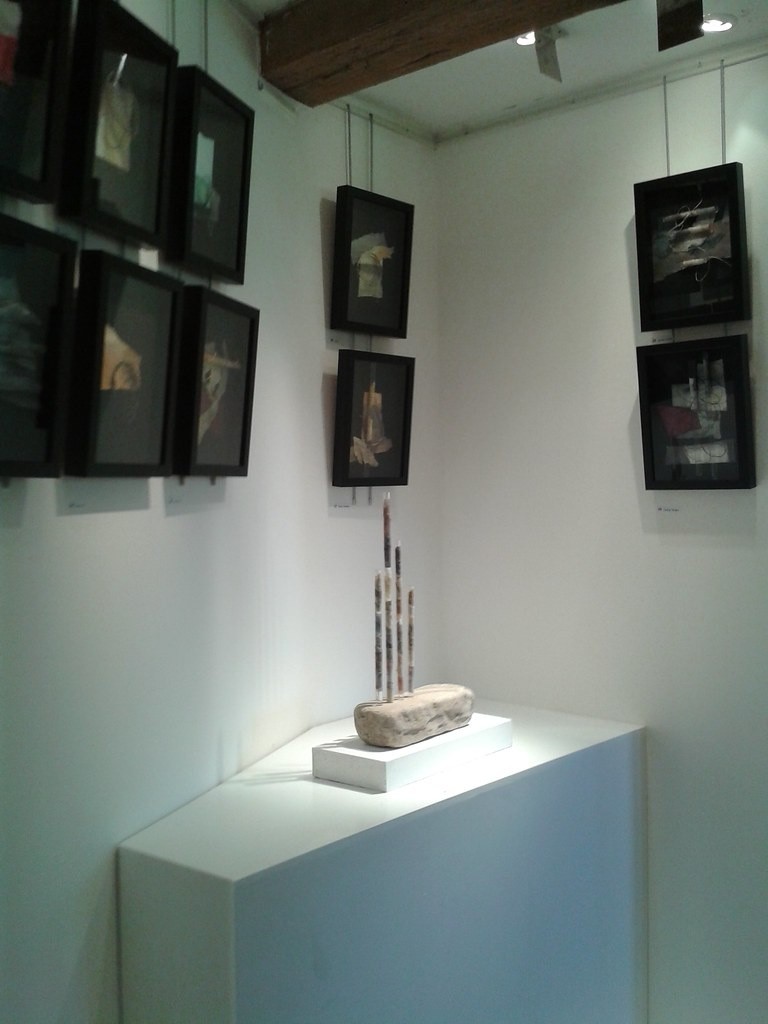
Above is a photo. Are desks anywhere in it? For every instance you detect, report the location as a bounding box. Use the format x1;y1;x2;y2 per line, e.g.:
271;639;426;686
117;696;646;1024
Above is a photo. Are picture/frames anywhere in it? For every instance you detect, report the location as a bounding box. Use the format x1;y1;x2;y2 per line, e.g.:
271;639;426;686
0;0;72;206
171;284;261;479
163;65;255;285
634;162;751;332
55;0;180;250
636;334;758;492
0;210;79;486
66;249;175;478
331;349;416;488
329;185;415;342
